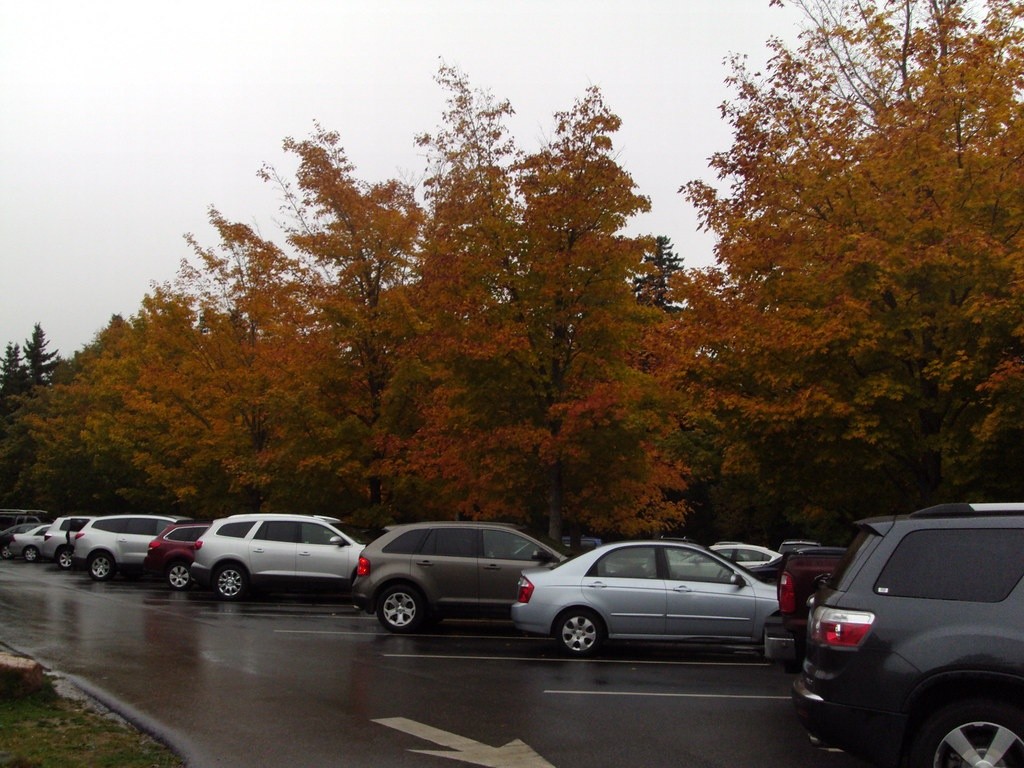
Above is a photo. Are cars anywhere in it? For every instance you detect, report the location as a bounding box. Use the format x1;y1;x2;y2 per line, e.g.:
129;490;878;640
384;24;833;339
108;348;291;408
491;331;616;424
512;541;785;656
0;524;49;560
680;541;783;571
10;525;52;561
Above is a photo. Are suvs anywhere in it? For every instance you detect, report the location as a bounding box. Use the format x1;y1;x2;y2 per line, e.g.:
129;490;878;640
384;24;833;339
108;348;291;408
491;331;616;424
792;500;1024;768
73;515;191;582
147;524;212;590
352;521;569;633
190;514;367;602
0;509;49;534
44;516;100;570
